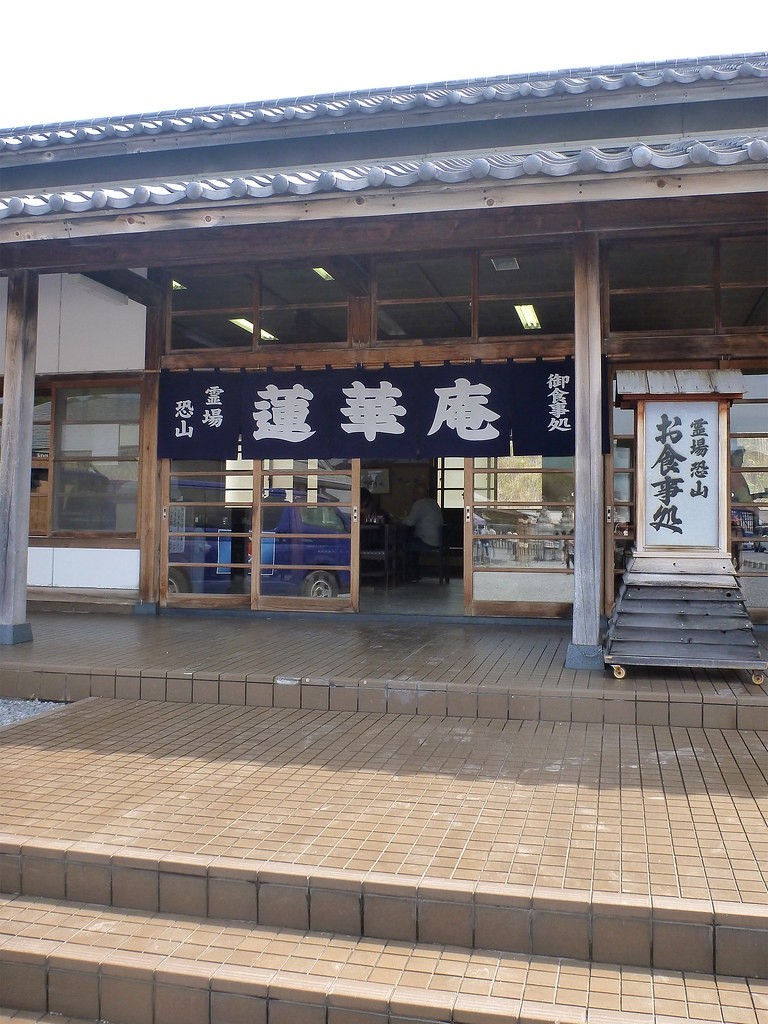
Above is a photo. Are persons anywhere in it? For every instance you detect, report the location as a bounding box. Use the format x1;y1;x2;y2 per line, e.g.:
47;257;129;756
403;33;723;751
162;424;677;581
565;529;575;568
400;483;443;583
481;525;496;556
361;486;373;508
507;529;518;555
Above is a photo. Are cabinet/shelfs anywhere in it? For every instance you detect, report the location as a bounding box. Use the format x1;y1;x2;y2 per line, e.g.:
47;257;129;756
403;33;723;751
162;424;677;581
360;523;397;592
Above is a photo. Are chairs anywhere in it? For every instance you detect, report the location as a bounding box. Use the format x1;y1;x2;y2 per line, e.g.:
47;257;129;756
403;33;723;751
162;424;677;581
414;524;449;585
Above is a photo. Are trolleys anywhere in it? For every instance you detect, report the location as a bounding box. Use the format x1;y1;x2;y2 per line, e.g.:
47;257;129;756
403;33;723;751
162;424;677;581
600;645;767;686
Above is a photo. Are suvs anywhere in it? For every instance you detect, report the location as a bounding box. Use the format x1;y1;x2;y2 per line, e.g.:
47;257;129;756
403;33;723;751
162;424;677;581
167;479;350;597
